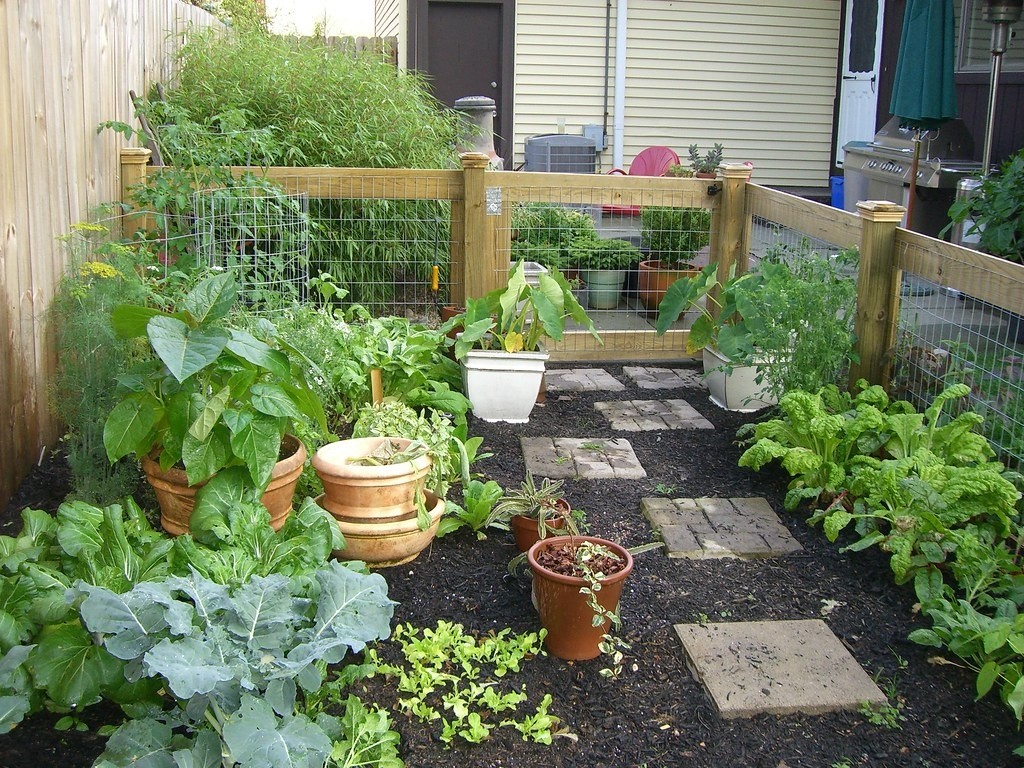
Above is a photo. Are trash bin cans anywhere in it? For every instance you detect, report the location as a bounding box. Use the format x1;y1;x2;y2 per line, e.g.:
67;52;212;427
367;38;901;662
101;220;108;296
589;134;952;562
832;176;845;210
940;178;1000;302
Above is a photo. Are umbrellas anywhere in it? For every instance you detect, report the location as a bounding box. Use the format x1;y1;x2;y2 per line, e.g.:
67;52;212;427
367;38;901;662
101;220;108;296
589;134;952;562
887;0;961;231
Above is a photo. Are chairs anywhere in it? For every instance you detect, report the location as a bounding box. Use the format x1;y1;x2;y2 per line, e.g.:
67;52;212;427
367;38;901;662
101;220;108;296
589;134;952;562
603;148;679;215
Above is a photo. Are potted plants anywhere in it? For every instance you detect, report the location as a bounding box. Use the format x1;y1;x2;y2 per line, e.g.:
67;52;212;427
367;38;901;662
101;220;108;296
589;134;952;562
440;261;605;423
659;164;696;178
637;205;710;318
689;143;725;177
563;236;645;309
482;471;571;552
308;433;471;570
658;260;794;410
105;274;328;541
523;537;634;682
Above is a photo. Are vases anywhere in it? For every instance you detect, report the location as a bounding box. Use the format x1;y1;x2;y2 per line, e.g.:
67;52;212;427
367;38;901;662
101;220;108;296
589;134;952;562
613;235;653;298
443;306;507;365
510;261;548;324
